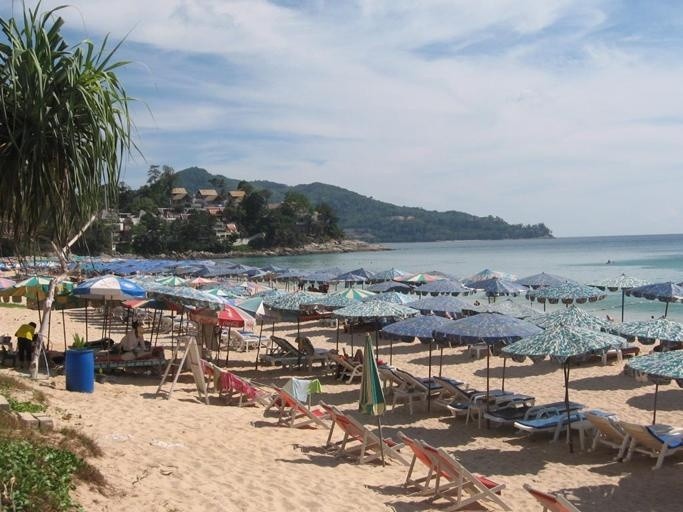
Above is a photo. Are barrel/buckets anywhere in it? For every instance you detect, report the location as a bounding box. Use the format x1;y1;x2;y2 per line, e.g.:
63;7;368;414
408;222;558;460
65;349;94;392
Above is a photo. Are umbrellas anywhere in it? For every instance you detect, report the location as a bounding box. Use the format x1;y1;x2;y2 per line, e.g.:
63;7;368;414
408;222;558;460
498;321;628;457
585;272;653;324
624;280;682;319
624;348;682;390
599;313;683;426
353;329;389;470
525;277;607;311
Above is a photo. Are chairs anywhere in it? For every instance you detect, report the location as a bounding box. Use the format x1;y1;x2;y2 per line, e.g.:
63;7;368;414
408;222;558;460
18;268;682;472
420;441;512;512
523;483;581;512
391;435;451;495
